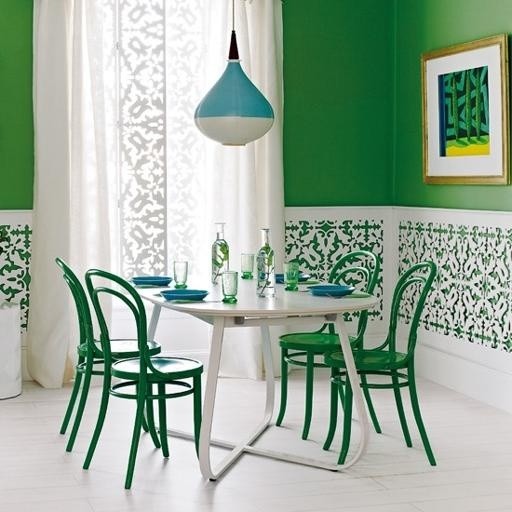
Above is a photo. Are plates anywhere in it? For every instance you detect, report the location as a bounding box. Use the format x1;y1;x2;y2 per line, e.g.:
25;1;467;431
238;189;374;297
273;272;312;285
307;284;355;297
159;289;209;301
131;276;172;286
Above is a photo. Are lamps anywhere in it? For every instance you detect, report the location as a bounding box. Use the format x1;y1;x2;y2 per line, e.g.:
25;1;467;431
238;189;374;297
191;0;277;148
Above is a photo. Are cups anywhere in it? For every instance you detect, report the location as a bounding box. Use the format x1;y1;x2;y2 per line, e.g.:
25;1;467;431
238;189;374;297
174;261;188;286
283;261;298;289
222;271;236;302
240;252;253;277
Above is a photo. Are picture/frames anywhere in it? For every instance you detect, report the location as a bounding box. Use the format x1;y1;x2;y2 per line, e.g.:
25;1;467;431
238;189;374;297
418;32;509;190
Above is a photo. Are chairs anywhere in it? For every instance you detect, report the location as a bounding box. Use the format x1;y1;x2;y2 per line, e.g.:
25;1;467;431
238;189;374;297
275;249;383;442
53;255;164;454
81;266;206;490
323;258;439;467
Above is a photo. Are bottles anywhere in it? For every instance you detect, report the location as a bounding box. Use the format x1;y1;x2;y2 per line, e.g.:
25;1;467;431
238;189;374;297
256;227;277;297
211;221;230;284
